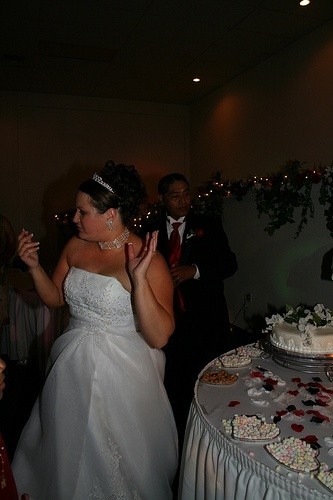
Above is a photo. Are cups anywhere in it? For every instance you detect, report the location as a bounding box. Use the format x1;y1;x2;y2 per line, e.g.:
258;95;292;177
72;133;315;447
168;263;177;287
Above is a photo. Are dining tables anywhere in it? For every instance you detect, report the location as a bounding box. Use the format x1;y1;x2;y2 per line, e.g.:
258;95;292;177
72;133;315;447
177;343;333;500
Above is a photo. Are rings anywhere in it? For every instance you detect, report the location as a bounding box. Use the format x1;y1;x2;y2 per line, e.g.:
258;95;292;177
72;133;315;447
153;252;157;254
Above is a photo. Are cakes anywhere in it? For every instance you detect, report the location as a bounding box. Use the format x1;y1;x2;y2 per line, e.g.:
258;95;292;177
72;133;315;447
270;302;333;353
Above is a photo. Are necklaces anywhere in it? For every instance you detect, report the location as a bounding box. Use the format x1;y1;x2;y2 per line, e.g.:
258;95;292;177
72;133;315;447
99;228;129;250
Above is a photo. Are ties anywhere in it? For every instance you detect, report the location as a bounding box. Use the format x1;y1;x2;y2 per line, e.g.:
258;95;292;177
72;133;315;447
168;222;183;274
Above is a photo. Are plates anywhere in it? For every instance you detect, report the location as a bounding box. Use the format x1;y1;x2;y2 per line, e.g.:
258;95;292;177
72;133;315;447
235;343;266;359
199;365;239;386
313;468;333;495
264;439;321;474
231;415;281;442
214;353;253;369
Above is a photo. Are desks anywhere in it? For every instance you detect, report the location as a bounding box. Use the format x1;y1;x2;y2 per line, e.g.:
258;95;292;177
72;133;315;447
0;266;71;376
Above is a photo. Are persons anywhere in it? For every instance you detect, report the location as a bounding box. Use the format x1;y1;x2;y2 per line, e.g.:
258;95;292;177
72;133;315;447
10;161;178;500
146;173;239;360
0;215;54;290
0;358;20;500
